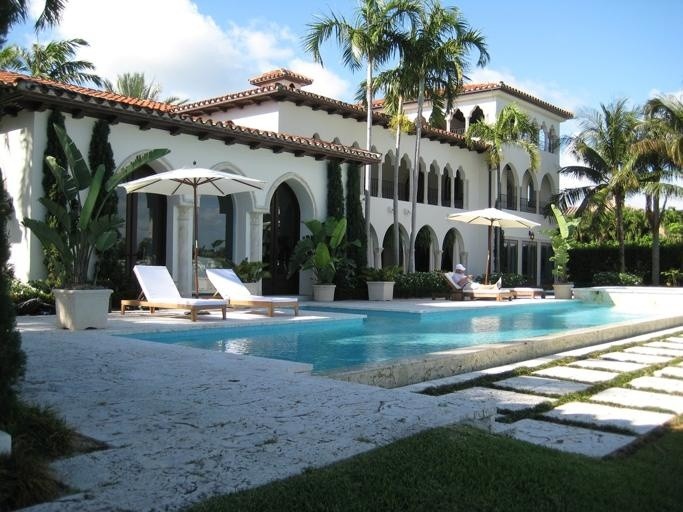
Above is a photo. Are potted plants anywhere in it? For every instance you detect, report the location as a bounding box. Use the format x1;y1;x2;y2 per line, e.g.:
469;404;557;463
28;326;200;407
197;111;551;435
367;263;403;301
543;204;583;300
20;120;169;332
291;216;362;302
229;258;272;296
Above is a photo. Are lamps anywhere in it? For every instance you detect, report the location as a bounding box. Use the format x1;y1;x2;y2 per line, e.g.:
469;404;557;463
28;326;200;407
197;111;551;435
528;231;534;241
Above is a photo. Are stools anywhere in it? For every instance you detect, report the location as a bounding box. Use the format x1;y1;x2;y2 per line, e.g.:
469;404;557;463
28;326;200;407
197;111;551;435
431;290;474;301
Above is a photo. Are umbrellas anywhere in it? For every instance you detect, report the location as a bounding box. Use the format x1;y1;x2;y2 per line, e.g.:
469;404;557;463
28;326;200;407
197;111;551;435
116;161;267;317
444;205;542;285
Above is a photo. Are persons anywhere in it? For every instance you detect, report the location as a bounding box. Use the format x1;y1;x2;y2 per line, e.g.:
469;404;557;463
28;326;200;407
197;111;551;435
451;262;503;291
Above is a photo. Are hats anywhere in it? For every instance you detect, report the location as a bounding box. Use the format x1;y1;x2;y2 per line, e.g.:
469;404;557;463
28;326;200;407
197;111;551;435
456;264;466;271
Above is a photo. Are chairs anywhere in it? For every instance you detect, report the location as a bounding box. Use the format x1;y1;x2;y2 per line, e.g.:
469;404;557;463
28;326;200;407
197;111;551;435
439;272;518;302
499;285;546;300
204;266;300;320
119;264;229;322
